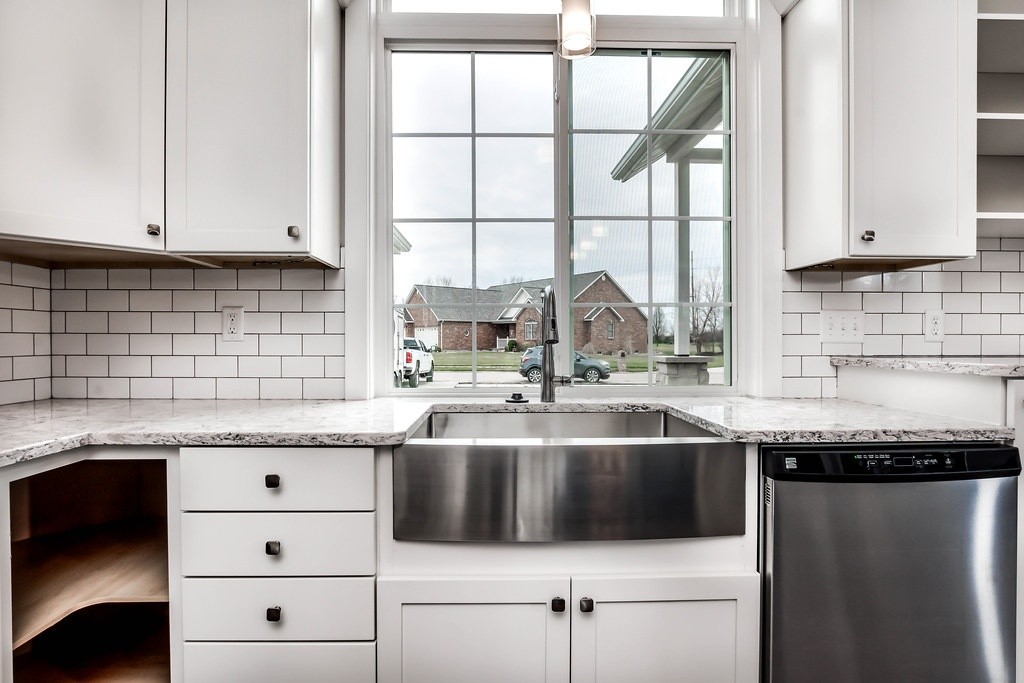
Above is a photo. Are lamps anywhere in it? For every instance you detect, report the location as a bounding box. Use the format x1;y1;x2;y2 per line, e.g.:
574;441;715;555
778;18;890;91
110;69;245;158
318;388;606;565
556;0;597;61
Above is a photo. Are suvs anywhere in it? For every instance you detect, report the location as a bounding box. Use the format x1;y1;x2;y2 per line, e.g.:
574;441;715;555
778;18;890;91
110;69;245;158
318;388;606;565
403;337;435;388
518;346;612;383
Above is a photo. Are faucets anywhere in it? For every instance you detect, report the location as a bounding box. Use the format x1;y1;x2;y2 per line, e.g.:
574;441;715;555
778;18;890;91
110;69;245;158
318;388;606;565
540;285;560;401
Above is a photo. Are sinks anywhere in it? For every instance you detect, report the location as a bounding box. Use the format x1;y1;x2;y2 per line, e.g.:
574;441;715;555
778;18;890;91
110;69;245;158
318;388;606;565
392;400;747;540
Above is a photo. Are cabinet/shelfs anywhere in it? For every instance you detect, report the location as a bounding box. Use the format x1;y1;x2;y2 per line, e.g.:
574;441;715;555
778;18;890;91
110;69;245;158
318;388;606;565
782;0;1024;272
0;446;763;683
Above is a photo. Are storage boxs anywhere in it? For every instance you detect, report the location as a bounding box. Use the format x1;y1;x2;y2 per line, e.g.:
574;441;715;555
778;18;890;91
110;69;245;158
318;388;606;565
0;0;342;270
